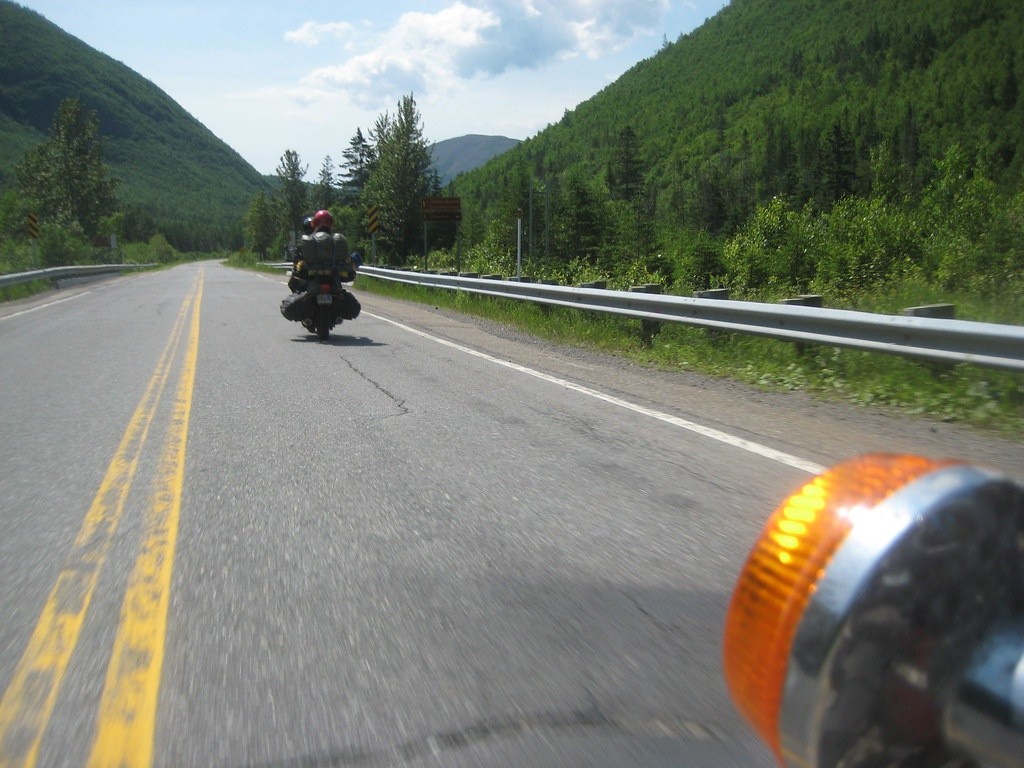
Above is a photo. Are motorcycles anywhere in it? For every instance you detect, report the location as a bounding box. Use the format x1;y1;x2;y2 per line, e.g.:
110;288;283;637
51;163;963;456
280;245;361;339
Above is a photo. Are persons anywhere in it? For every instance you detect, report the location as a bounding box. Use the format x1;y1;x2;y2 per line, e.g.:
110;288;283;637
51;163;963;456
292;210;362;283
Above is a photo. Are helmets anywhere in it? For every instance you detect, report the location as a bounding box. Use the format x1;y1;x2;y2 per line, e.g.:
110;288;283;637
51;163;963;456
303;216;314;235
312;209;334;227
